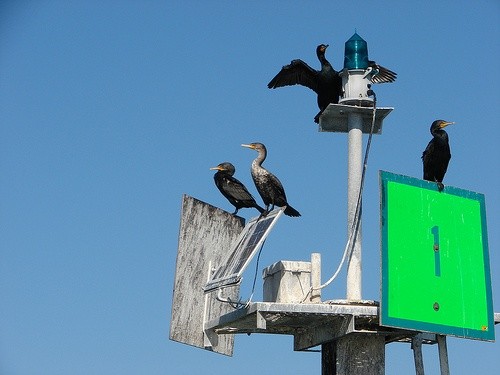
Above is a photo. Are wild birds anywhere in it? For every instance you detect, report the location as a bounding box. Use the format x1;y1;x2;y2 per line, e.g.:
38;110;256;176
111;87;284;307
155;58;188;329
421;120;456;191
209;162;265;215
267;44;397;124
241;143;302;224
